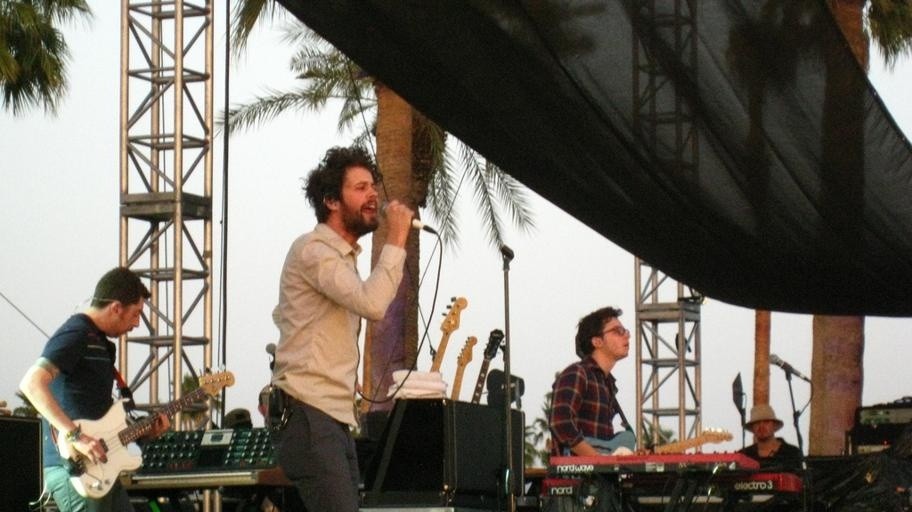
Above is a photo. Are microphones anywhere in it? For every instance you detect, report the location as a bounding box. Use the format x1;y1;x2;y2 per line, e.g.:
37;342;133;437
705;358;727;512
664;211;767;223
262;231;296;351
768;353;811;384
381;201;438;240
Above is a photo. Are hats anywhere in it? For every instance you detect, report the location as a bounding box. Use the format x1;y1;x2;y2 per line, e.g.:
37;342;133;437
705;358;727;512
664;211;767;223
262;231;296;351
743;404;784;433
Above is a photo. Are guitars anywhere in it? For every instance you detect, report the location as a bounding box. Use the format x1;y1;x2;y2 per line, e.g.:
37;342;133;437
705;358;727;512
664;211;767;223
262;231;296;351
564;428;733;494
429;297;506;405
58;364;235;499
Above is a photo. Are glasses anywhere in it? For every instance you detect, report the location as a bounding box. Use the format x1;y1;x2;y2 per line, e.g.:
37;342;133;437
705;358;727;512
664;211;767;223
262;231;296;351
603;325;630;335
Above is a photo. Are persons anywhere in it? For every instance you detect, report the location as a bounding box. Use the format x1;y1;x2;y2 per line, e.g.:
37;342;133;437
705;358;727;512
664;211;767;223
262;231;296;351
20;267;153;512
266;145;416;512
547;307;631;456
736;403;802;473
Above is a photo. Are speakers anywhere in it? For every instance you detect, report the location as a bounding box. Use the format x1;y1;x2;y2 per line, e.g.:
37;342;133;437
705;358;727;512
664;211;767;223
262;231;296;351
382;395;525;508
0;414;44;512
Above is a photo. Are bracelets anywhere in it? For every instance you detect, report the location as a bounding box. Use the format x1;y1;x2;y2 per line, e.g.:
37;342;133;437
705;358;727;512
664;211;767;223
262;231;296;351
65;425;82;442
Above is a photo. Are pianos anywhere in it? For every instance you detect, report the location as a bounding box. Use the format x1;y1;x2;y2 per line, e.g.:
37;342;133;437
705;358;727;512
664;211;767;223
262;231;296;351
541;452;760;478
544;473;804;494
